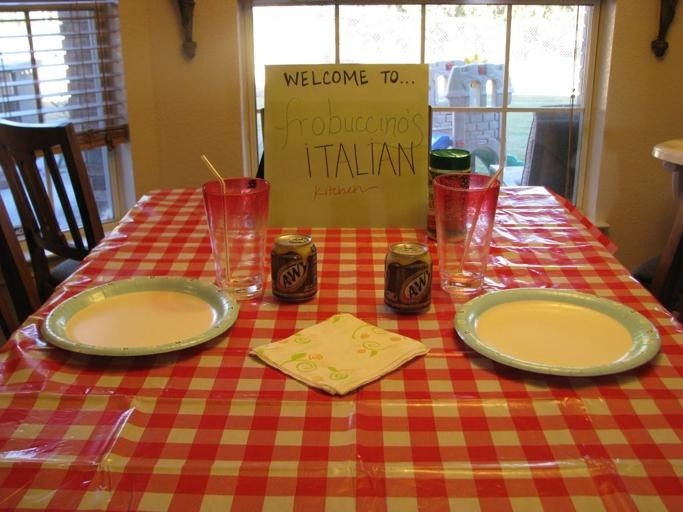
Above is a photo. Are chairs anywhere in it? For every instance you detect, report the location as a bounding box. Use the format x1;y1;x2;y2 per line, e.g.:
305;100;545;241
0;118;105;341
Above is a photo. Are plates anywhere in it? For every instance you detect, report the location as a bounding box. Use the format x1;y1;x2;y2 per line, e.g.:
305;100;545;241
40;274;240;358
452;288;661;377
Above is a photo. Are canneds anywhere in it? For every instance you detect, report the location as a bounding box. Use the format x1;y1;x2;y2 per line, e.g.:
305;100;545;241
383;241;433;316
270;233;318;302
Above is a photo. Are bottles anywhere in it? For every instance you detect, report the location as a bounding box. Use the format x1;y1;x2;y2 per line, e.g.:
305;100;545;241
426;148;469;243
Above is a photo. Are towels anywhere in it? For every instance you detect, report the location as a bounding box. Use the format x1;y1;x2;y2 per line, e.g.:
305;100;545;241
250;313;430;398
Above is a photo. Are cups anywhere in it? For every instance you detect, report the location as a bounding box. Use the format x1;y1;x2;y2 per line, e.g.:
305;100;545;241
434;173;501;297
202;176;270;299
